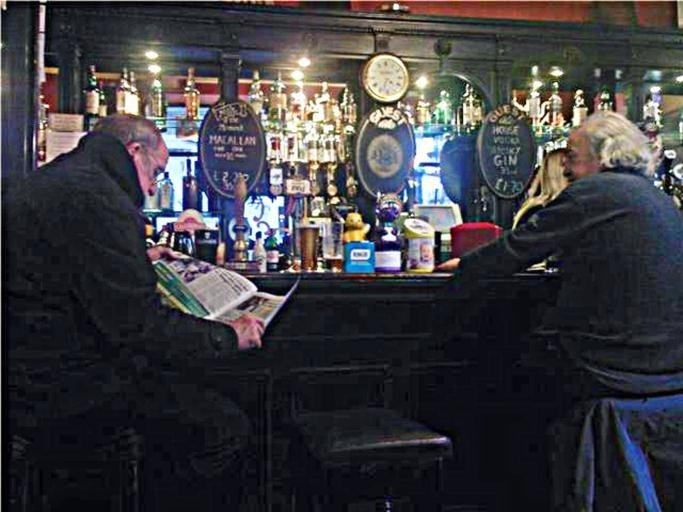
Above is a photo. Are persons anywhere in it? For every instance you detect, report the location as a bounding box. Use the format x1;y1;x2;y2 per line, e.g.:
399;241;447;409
2;112;268;511
430;111;683;512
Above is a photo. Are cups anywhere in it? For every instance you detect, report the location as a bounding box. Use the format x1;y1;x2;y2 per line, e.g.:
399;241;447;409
194;228;219;266
319;220;345;272
296;225;319;272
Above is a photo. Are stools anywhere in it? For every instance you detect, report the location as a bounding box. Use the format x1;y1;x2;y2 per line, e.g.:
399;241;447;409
17;429;146;512
288;407;454;512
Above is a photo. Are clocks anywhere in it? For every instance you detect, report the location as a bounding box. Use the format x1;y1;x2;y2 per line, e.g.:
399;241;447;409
361;52;411;106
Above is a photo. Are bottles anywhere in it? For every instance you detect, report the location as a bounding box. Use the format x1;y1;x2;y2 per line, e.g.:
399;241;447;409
521;79;683;211
253;228;280;274
79;64;358;216
